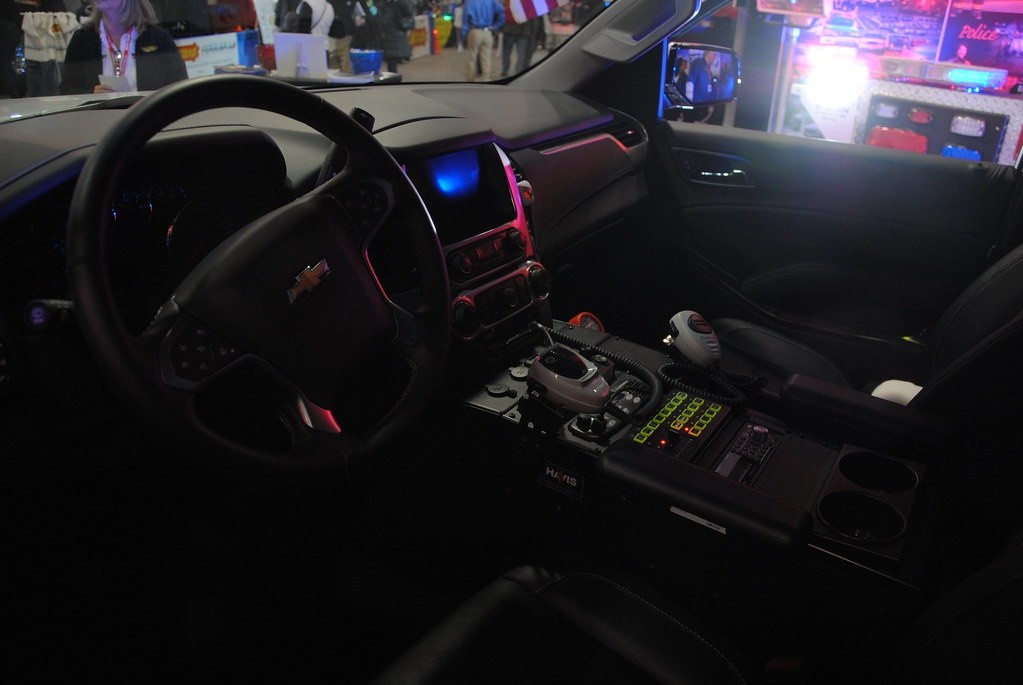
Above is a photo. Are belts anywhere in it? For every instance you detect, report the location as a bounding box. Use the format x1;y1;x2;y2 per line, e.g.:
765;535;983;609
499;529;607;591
470;25;490;31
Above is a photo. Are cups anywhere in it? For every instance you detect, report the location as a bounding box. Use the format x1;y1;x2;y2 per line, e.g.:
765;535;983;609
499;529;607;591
274;34;301;77
568;312;604;333
297;35;328;80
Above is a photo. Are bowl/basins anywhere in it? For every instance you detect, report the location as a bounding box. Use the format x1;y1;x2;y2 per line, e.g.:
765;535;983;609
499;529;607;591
256;43;276;71
348;49;383;75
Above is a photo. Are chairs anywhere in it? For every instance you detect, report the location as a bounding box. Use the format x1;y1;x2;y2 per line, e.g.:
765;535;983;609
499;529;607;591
366;554;1023;685
714;242;1023;393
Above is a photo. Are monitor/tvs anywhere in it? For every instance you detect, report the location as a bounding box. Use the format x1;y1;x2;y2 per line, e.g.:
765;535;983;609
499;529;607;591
403;143;517;246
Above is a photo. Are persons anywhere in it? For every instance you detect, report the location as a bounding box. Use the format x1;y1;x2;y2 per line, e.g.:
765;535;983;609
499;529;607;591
274;0;415;73
949;43;972;65
674;49;733;102
454;0;540;81
569;0;606;33
0;0;188;95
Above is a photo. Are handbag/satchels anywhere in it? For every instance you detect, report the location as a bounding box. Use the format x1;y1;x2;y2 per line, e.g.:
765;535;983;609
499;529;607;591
330;16;346;38
395;0;415;31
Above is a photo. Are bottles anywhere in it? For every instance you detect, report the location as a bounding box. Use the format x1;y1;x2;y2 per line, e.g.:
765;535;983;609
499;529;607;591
16;48;24;74
